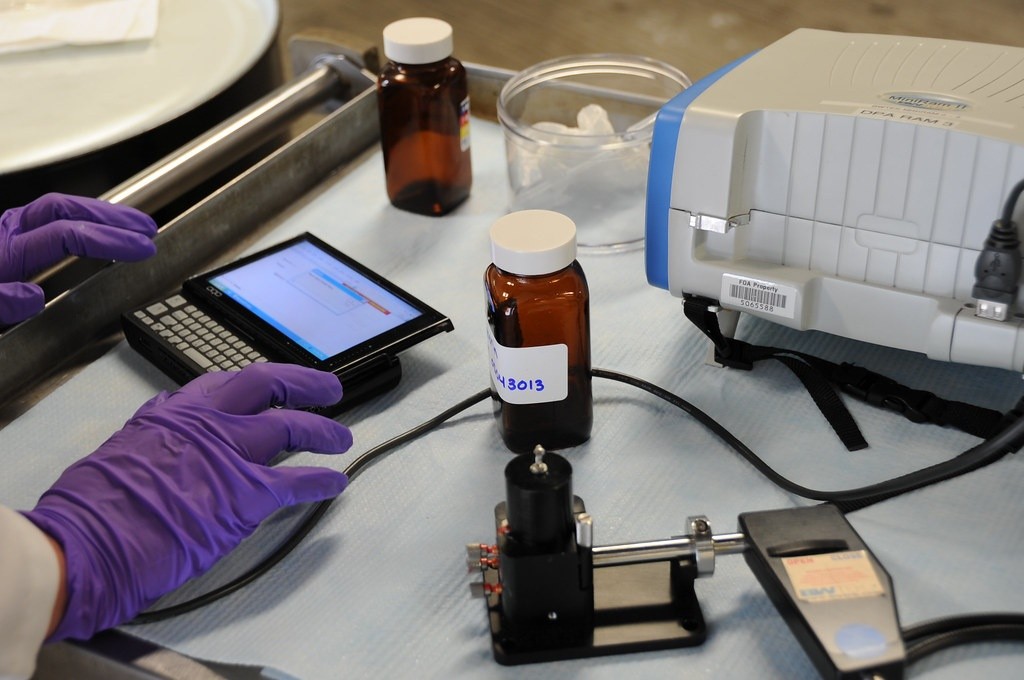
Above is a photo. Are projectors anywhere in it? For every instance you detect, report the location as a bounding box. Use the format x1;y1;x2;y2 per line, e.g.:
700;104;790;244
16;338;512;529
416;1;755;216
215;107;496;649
644;26;1024;374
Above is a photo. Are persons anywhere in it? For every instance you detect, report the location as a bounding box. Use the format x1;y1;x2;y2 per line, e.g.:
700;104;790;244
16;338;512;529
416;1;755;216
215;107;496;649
0;192;353;680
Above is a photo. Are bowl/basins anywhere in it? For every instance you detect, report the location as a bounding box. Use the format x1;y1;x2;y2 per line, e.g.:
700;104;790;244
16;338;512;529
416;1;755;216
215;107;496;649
496;54;692;251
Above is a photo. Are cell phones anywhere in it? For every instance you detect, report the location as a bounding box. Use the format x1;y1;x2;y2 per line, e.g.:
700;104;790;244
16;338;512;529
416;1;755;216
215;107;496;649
123;231;455;420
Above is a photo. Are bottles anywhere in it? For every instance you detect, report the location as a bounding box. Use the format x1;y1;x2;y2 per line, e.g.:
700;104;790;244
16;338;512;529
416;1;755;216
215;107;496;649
484;210;593;454
377;15;473;216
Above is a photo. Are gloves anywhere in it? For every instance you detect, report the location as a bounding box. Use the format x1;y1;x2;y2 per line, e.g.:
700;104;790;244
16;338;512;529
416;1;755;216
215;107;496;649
15;362;355;646
0;192;159;330
